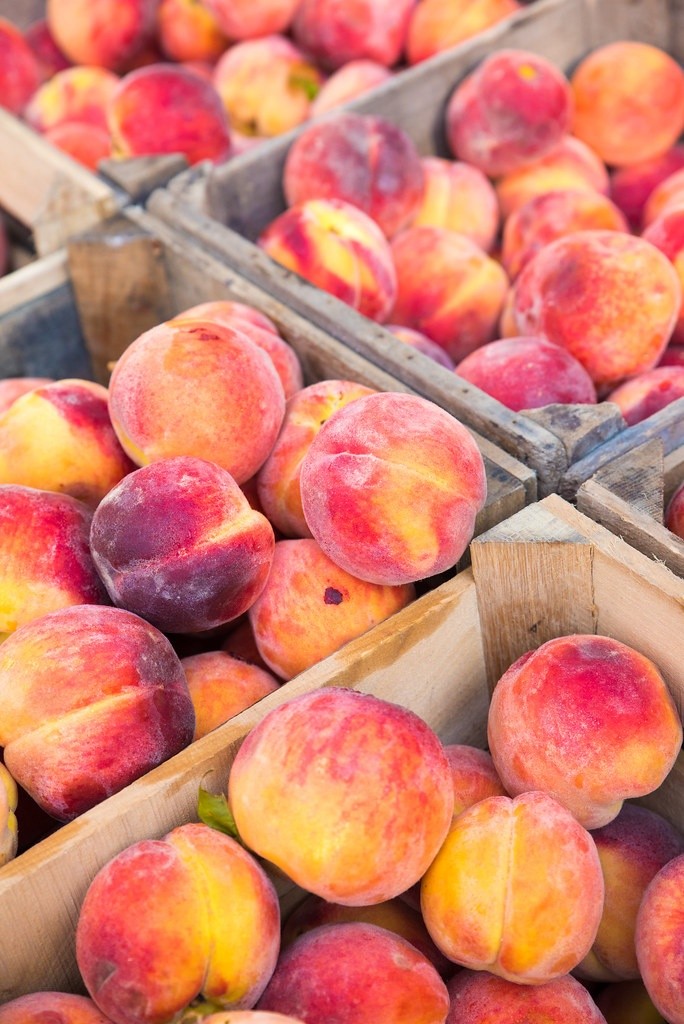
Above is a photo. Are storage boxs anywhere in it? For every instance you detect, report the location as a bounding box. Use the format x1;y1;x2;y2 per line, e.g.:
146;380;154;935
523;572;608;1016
2;1;684;1002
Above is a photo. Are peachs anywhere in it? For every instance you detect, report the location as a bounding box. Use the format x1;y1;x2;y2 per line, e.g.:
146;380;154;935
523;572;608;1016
0;297;684;1024
0;0;684;424
